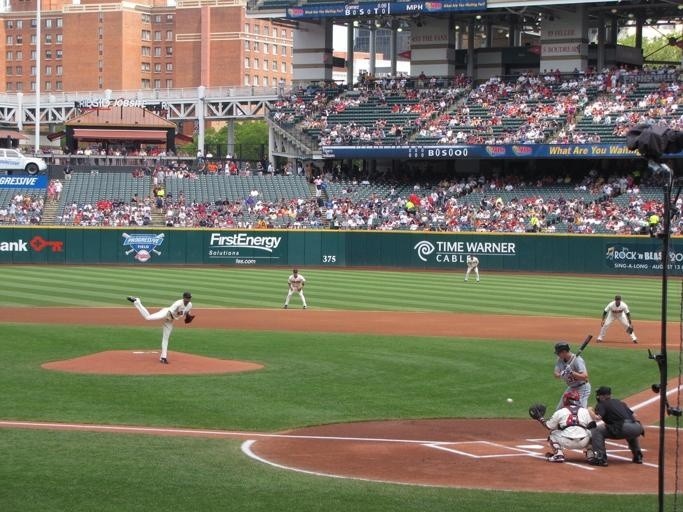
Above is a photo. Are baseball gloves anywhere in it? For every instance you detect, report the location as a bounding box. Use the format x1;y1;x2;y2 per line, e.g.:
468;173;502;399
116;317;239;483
528;405;546;421
627;324;635;333
298;285;301;292
184;314;193;323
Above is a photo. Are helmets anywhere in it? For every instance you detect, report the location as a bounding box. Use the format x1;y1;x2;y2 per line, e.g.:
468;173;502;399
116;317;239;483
596;387;610;401
555;343;570;354
563;391;579;405
183;291;192;298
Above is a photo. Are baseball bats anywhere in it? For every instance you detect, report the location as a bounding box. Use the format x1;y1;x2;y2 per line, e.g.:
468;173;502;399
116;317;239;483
561;333;592;376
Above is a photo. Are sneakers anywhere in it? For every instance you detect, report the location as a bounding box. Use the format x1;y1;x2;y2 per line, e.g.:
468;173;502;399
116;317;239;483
161;358;168;364
634;454;642;463
127;296;136;302
549;454;564;462
588;456;608;466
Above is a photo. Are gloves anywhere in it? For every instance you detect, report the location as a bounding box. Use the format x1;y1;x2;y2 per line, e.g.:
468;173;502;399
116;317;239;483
561;365;571;377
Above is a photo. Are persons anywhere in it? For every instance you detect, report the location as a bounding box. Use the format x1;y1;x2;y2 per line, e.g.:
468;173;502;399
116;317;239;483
1;64;683;238
553;342;591;409
596;295;639;344
528;389;597;463
127;292;193;364
284;269;307;309
587;385;644;466
464;254;480;282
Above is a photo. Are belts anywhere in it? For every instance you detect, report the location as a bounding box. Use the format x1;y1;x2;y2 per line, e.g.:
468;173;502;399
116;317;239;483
571;380;587;388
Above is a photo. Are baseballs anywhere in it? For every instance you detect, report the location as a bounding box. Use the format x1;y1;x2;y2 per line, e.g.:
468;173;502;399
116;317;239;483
507;398;512;403
